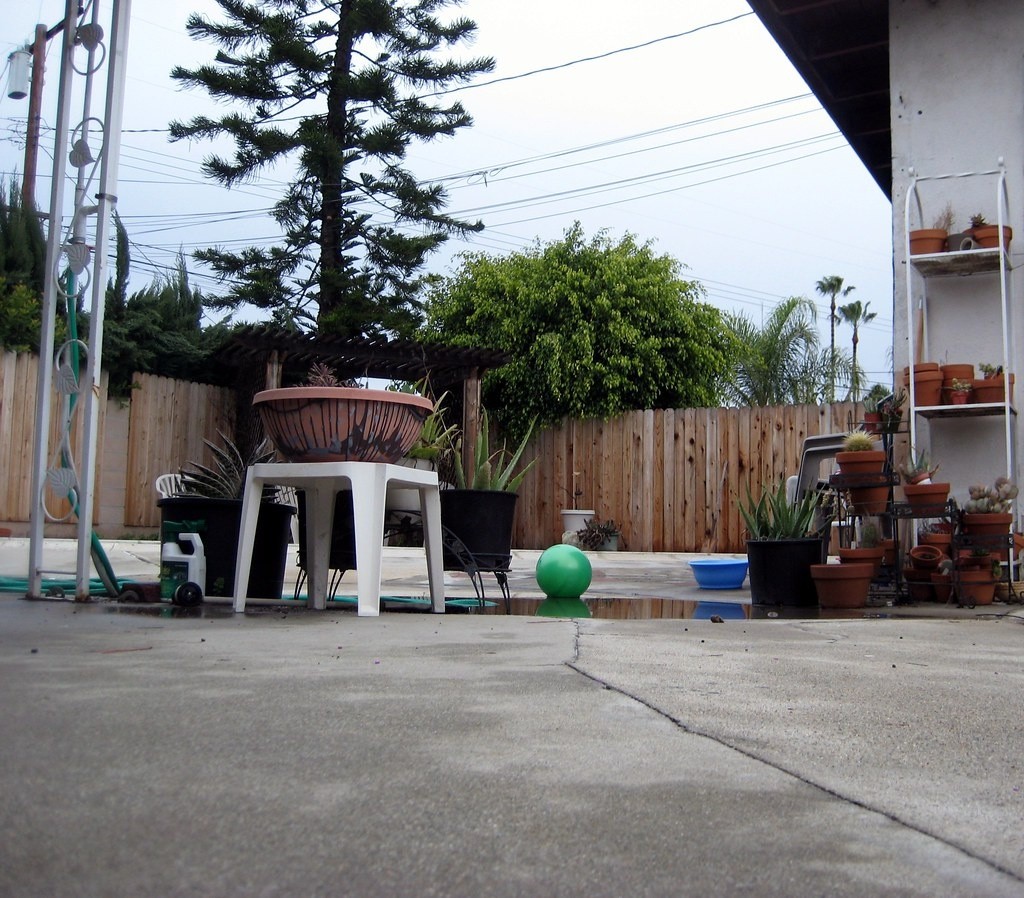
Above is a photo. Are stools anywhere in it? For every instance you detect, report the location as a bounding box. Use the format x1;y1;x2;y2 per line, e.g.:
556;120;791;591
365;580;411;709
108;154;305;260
231;462;446;617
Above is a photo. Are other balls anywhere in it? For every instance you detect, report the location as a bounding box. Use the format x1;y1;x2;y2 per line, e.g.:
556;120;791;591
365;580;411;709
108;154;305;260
535;598;592;618
535;545;593;597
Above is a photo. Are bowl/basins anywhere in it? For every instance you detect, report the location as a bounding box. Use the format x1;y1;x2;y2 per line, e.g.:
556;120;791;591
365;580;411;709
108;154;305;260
689;559;749;589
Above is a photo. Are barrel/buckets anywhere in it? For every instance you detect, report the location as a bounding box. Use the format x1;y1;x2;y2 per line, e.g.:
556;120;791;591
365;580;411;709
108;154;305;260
160;532;206;601
560;510;595;532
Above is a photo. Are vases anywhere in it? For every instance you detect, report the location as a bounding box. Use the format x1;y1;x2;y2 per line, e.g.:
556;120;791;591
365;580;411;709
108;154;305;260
900;483;951;514
946;233;973;251
1013;532;1024;562
903;546;943;601
847;475;891;514
903;363;974;407
810;563;875;609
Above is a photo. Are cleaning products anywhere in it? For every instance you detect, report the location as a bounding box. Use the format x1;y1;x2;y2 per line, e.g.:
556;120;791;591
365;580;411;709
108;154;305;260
158;518;207;604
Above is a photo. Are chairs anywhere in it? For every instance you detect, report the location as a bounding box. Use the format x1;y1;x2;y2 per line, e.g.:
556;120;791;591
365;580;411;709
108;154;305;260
274;484;300;545
784;390;893;546
156;474;196;498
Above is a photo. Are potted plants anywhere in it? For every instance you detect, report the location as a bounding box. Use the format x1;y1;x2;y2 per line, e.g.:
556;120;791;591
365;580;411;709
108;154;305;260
862;383;907;431
973;363;1014;403
733;469;830;608
909;229;948;254
576;518;622;551
896;441;941;485
919;477;1018;604
838;522;895;577
963;213;1012;254
835;430;886;473
935;378;973;404
156;364;541;614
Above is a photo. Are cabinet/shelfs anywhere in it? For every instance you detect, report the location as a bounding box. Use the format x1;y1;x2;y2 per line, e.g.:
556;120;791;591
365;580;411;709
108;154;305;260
892;498;1024;610
828;409;910;609
904;155;1024;582
